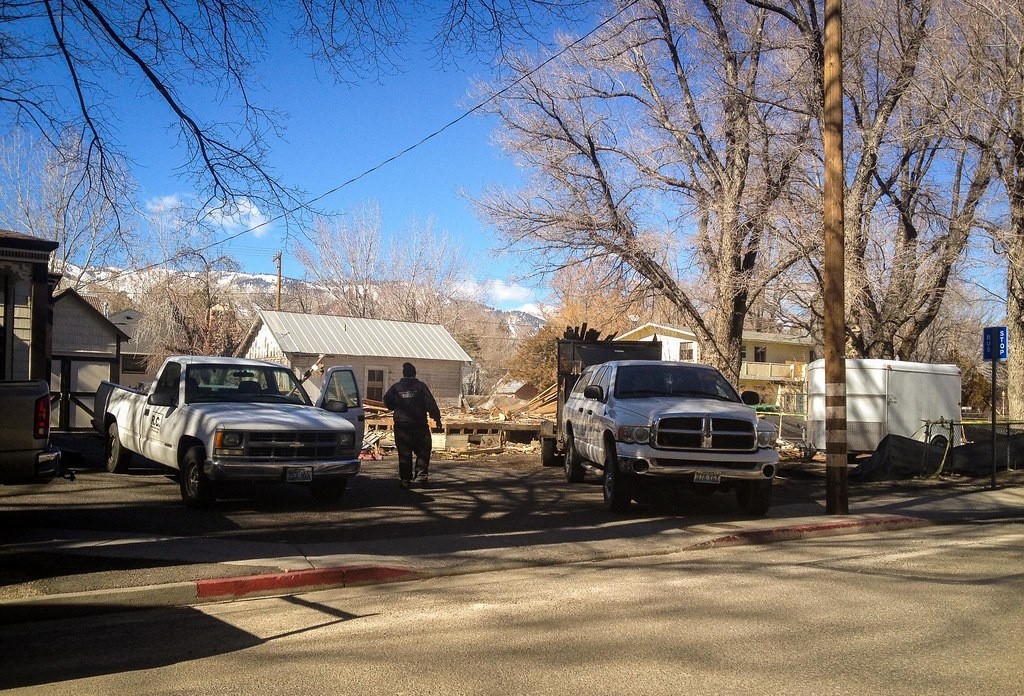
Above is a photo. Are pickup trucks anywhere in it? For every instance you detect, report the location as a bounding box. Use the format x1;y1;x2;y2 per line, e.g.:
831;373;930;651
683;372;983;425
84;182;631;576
89;355;366;516
0;379;62;486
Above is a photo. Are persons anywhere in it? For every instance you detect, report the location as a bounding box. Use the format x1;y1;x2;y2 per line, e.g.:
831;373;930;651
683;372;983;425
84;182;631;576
383;362;441;488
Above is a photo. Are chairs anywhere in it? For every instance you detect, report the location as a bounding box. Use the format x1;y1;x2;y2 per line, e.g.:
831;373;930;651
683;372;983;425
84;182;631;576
173;377;263;395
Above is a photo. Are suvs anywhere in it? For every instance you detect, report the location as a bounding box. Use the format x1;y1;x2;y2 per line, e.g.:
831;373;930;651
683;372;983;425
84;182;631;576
559;359;781;518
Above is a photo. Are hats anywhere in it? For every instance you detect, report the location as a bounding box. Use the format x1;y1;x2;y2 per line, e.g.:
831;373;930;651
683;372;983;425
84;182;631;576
402;362;416;377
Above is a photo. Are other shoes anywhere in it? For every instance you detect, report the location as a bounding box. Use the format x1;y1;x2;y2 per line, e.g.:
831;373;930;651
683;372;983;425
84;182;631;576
416;482;429;488
401;482;415;490
